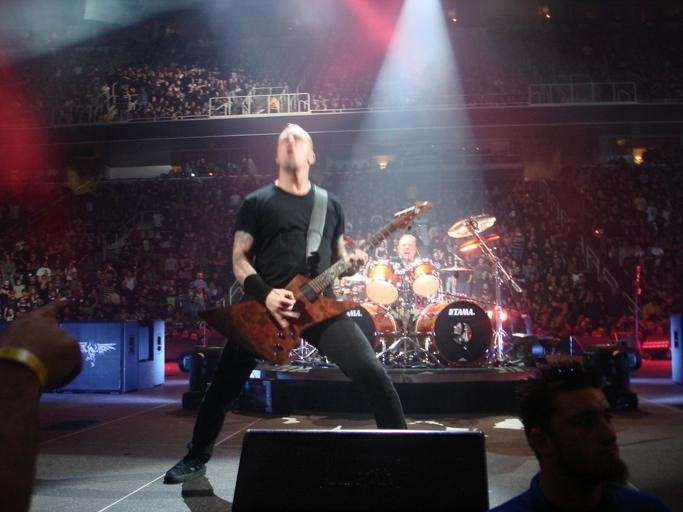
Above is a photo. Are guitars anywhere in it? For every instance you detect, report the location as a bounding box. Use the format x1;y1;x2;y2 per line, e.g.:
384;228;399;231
197;200;433;369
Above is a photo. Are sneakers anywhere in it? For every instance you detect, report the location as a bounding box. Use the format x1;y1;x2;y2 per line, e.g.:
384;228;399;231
164;454;206;482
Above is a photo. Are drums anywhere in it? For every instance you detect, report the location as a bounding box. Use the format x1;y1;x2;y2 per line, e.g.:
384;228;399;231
414;299;494;366
365;263;399;304
344;301;397;351
412;263;441;300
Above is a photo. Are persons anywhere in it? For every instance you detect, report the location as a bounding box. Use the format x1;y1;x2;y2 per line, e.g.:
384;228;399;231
0;297;82;512
2;38;682;342
490;361;670;512
162;124;407;483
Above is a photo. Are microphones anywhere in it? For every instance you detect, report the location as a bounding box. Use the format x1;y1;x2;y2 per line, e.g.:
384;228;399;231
463;212;482;233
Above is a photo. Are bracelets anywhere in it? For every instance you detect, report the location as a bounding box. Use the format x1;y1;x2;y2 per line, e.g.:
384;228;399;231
0;346;47;393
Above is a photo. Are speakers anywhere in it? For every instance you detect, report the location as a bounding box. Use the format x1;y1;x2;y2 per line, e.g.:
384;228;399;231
229;428;491;511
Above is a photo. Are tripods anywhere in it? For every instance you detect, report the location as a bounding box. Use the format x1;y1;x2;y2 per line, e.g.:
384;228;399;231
283;337;322;372
470;269;526;371
376;286;441;369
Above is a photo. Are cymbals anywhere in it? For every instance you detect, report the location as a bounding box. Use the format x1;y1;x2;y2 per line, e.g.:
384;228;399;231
458;233;500;252
447;214;497;239
441;266;473;273
